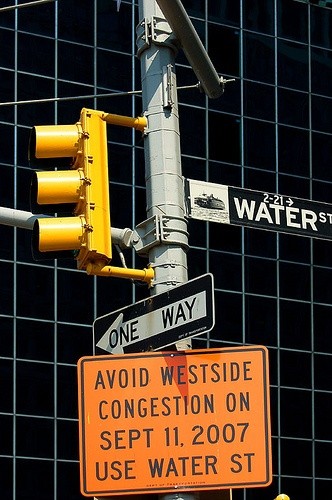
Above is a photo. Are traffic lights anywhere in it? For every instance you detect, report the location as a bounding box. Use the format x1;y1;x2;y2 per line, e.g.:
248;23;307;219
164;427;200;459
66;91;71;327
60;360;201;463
27;107;111;269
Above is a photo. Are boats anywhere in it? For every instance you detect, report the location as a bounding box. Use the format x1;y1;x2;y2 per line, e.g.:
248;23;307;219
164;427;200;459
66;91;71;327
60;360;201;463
193;194;224;209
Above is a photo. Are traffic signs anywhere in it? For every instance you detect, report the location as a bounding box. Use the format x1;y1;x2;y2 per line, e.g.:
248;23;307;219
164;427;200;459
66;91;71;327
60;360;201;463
76;345;272;497
184;178;331;241
92;273;215;356
